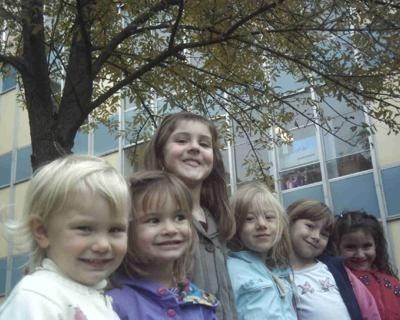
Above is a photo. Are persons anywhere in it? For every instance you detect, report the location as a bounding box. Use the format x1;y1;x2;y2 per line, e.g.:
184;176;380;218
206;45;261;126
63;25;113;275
144;110;240;320
223;181;300;320
332;209;400;320
104;170;220;320
0;153;132;320
278;198;381;320
